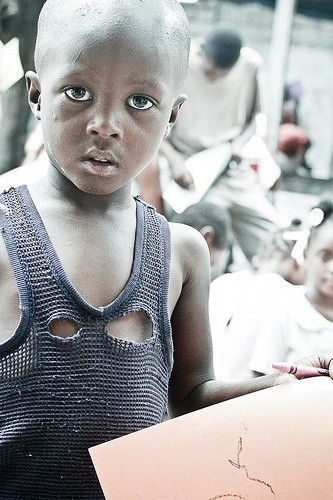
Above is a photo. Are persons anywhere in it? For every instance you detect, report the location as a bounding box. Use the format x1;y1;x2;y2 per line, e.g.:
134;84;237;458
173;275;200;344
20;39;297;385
165;203;231;266
208;202;333;381
133;29;282;271
269;124;310;175
0;0;333;500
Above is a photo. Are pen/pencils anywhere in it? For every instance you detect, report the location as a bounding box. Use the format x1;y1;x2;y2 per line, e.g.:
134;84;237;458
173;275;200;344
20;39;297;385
270;360;329;378
